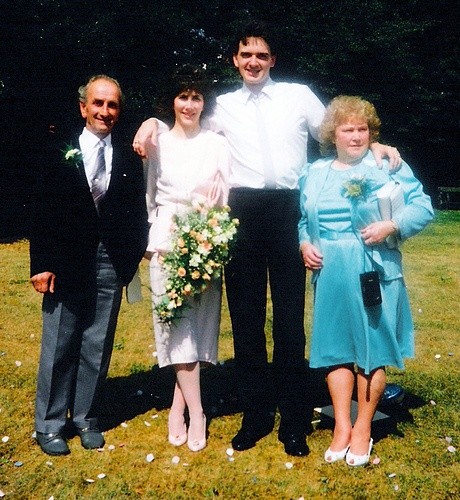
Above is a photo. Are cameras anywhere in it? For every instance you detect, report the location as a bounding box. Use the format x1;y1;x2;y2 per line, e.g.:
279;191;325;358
359;271;382;307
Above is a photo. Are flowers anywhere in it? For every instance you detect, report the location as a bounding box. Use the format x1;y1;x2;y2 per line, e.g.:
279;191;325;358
342;178;370;203
61;144;82;170
150;202;240;328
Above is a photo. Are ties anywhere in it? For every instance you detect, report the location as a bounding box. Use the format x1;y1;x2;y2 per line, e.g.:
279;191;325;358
92;140;107;210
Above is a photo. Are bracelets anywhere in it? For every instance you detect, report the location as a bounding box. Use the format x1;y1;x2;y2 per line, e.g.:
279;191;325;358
389;219;396;234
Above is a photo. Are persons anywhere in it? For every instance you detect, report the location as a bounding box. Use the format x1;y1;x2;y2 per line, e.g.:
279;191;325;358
146;60;230;452
30;74;150;456
133;18;402;458
298;96;434;466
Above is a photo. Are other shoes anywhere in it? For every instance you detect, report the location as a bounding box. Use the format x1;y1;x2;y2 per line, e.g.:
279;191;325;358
346;437;373;466
168;417;187;446
325;442;351;463
188;413;207;452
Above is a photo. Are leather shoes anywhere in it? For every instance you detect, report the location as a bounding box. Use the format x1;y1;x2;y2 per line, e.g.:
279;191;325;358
36;431;68;456
78;426;104;450
232;427;273;451
278;431;309;456
